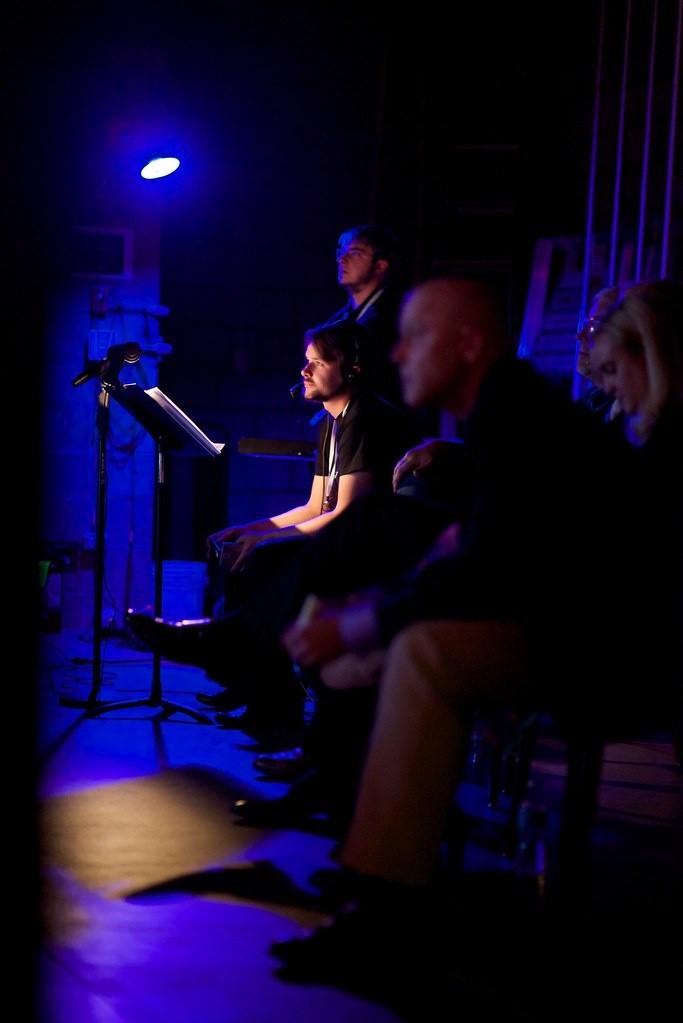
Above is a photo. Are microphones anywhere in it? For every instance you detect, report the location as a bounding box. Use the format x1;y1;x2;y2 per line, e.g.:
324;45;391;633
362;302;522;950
290;382;308;398
71;341;141;386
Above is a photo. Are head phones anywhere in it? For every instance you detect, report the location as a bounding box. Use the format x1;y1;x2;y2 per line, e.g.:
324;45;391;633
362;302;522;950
346;329;361;381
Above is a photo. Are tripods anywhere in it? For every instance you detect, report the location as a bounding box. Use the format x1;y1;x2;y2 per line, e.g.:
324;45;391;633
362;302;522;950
83;388;217;727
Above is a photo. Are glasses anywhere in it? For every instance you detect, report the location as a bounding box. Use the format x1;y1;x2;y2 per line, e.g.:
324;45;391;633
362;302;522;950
578;317;615;333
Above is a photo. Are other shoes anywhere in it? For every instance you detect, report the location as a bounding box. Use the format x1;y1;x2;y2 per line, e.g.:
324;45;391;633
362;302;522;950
126;606;257;687
196;680;464;989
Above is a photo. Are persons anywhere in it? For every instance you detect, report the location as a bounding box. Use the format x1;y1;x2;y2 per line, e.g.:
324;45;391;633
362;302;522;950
123;220;680;988
